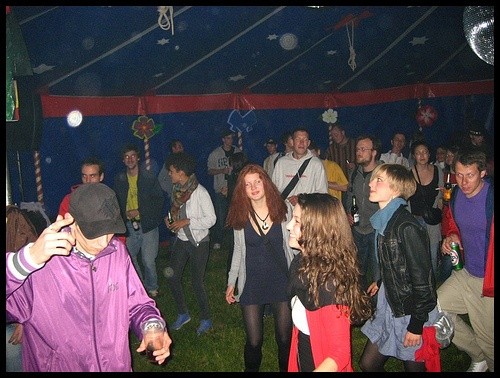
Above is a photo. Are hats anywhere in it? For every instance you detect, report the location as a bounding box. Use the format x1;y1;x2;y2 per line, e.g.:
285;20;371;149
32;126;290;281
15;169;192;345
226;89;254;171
69;182;126;239
470;123;486;136
266;136;278;145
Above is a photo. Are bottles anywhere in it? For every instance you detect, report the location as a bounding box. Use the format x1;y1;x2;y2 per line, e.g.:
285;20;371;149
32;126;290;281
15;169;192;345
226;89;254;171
131;217;139;231
442;173;452;204
225;164;228;180
351;195;359;226
168;209;175;235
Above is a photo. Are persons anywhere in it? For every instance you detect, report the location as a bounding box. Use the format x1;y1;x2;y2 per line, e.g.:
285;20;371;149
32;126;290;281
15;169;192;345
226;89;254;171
436;150;495;372
208;129;240;251
285;192;357;372
7;205;38;372
160;138;192;200
259;122;497;327
6;183;173;373
113;145;161;296
165;158;217;337
359;165;439;372
58;157;125;248
226;165;293;372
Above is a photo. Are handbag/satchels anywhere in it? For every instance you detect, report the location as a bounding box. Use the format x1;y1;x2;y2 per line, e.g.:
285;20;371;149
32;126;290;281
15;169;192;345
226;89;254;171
423;208;443;226
434;312;455;348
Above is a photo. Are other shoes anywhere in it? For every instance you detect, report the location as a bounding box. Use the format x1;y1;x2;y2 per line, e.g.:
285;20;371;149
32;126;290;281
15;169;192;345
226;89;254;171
213;243;221;250
147;290;158;298
172;312;191;330
197;317;212;334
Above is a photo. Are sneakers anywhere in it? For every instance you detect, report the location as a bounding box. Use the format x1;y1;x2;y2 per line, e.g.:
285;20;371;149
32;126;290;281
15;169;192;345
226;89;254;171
467;360;488;372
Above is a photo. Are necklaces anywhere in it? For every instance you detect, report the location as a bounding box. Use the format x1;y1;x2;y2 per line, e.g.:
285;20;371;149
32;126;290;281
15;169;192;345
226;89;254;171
254;210;271;230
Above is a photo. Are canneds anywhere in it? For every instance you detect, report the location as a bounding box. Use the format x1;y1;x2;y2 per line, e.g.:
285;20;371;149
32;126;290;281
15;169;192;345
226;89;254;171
449;242;463;271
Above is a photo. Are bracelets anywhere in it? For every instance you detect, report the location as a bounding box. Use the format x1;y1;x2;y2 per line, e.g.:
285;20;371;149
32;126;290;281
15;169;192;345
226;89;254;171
145;323;162;329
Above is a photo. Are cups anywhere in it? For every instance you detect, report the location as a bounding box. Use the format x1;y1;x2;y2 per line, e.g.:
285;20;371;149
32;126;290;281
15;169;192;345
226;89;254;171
140;319;166;362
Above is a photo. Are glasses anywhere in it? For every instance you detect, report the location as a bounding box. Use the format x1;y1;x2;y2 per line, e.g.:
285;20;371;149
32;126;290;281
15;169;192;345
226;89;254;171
123;153;138;159
356;148;374;151
